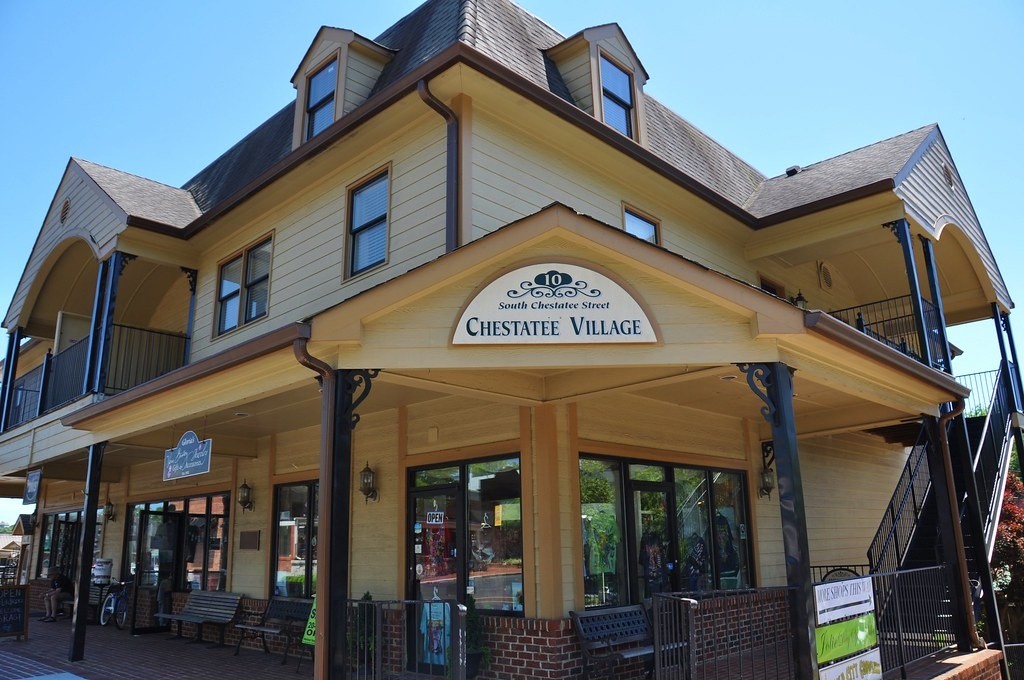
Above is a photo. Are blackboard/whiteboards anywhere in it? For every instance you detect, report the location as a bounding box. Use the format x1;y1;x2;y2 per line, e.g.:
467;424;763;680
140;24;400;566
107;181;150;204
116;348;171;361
0;585;30;637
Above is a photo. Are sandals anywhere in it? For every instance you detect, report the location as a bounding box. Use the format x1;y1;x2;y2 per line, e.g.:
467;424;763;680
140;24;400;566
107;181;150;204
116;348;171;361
43;616;57;622
37;616;49;621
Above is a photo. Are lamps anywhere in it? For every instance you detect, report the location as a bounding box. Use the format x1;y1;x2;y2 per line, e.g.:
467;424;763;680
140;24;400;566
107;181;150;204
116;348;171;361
790;289;808;311
758;461;776;498
238;479;254;514
358;461;378;507
102;498;116;524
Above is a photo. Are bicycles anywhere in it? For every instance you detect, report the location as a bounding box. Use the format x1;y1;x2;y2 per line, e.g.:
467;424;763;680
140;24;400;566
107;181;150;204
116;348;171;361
99;577;136;630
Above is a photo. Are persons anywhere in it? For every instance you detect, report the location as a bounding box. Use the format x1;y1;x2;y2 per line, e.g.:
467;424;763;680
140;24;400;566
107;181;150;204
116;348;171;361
37;566;73;622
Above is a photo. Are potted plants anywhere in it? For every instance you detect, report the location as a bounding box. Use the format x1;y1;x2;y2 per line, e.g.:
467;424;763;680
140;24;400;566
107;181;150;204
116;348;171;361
347;590;385;676
446;595;492;679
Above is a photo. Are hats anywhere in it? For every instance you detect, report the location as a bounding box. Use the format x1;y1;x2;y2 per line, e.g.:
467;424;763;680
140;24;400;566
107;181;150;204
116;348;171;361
47;567;59;575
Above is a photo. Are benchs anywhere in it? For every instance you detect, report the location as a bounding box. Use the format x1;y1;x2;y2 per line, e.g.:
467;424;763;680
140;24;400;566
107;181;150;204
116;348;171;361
153;589;245;649
569;599;689;680
58;585;109;619
234;595;315;673
